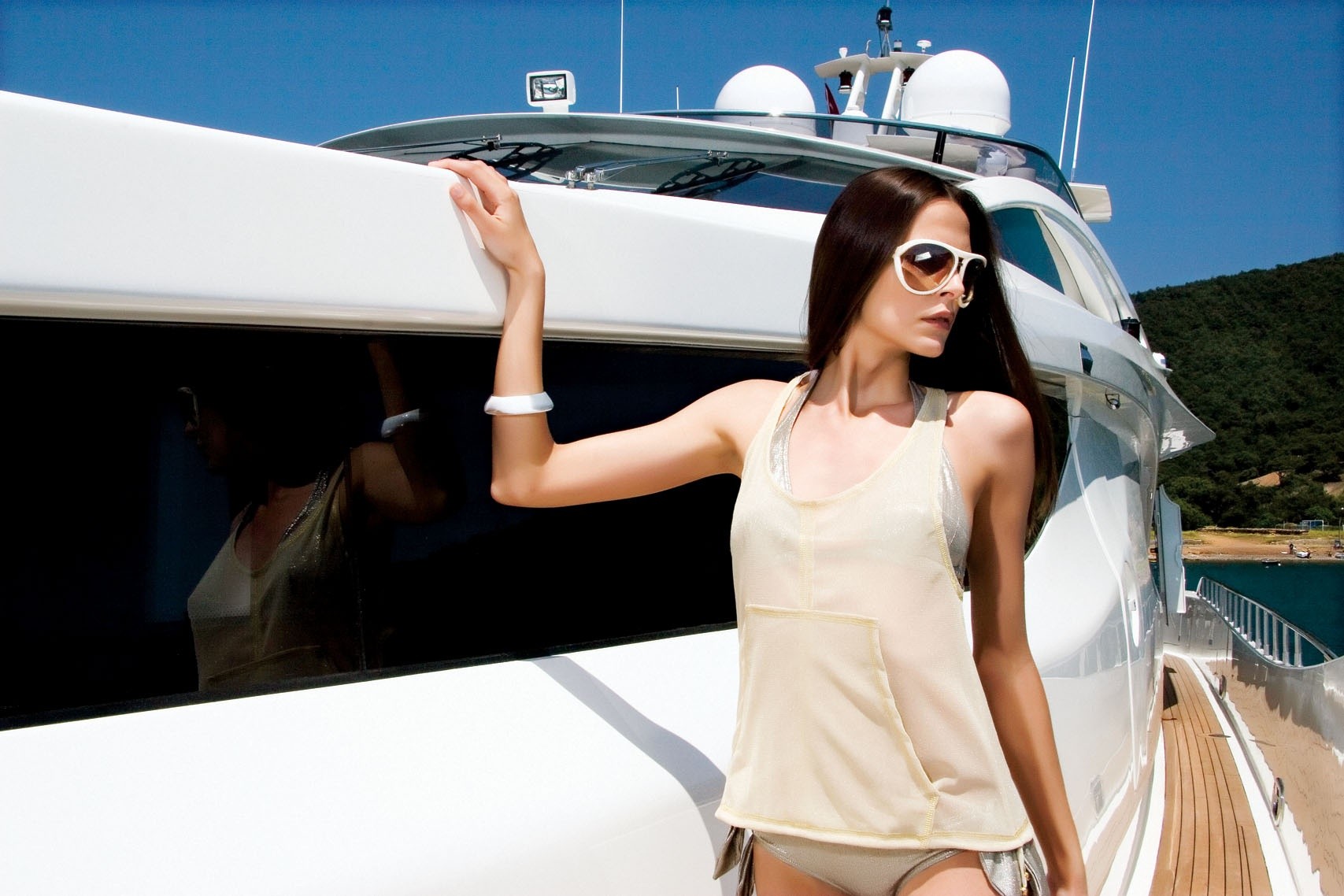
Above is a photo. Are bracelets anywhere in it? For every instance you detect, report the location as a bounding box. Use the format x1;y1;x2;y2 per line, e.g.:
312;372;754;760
381;409;418;437
484;391;554;416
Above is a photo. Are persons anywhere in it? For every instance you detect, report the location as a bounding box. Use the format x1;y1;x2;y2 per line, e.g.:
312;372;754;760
1306;547;1311;558
428;159;1088;896
188;342;429;695
1289;543;1297;555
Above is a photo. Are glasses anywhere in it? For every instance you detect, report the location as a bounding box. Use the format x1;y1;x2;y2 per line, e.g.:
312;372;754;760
894;240;989;309
